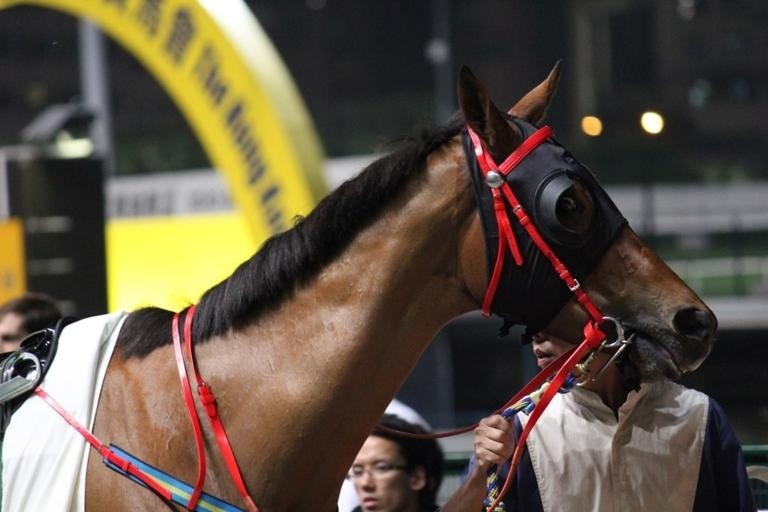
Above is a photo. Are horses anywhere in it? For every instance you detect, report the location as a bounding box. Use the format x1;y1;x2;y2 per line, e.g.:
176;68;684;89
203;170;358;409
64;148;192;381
0;58;719;510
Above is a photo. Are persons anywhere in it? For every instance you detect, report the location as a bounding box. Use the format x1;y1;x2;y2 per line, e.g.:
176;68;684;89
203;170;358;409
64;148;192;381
351;413;445;512
0;297;61;355
441;331;760;512
